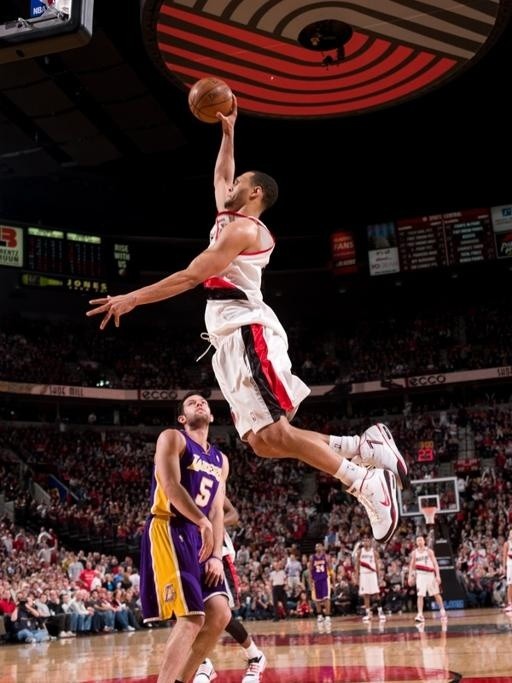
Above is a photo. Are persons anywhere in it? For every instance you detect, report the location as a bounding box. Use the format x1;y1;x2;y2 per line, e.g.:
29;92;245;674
268;562;290;621
36;425;156;471
308;543;332;622
456;402;510;470
2;334;119;388
233;543;313;620
313;645;337;683
355;538;385;619
117;341;192;389
372;413;459;510
192;496;271;682
391;305;509;377
84;74;408;546
270;646;295;682
407;536;446;621
352;644;396;682
301;501;436;616
1;513;171;643
293;416;369;511
301;321;395;386
435;470;510;616
34;468;151;544
207;432;307;547
139;391;234;682
411;621;452;681
3;424;38;516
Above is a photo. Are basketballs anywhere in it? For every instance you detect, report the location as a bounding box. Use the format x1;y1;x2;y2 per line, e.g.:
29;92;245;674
188;77;233;122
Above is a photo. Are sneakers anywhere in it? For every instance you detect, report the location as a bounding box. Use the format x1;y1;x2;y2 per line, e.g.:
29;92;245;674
59;630;76;637
317;614;331;624
193;658;217;683
345;423;408;543
440;608;445;615
363;613;373;619
378;612;385;618
415;614;425;621
242;650;267;683
503;605;512;611
